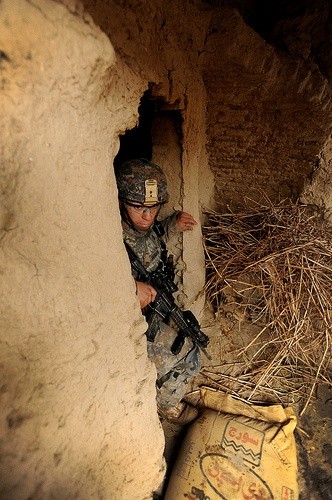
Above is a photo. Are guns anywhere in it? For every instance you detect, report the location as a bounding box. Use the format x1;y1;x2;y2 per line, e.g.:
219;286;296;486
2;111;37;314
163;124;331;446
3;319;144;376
122;235;213;361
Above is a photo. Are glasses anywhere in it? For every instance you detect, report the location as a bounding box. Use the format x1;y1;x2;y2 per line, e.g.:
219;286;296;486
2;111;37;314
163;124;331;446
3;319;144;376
123;201;162;214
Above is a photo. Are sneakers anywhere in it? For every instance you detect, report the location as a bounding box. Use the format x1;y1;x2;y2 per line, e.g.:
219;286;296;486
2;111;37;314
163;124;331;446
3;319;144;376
164;401;199;426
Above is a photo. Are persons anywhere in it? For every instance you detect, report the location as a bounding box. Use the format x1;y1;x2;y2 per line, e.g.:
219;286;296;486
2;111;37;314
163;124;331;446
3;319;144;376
114;158;198;427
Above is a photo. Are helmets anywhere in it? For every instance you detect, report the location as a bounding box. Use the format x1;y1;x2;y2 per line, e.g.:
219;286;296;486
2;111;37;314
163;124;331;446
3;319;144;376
117;160;169;208
